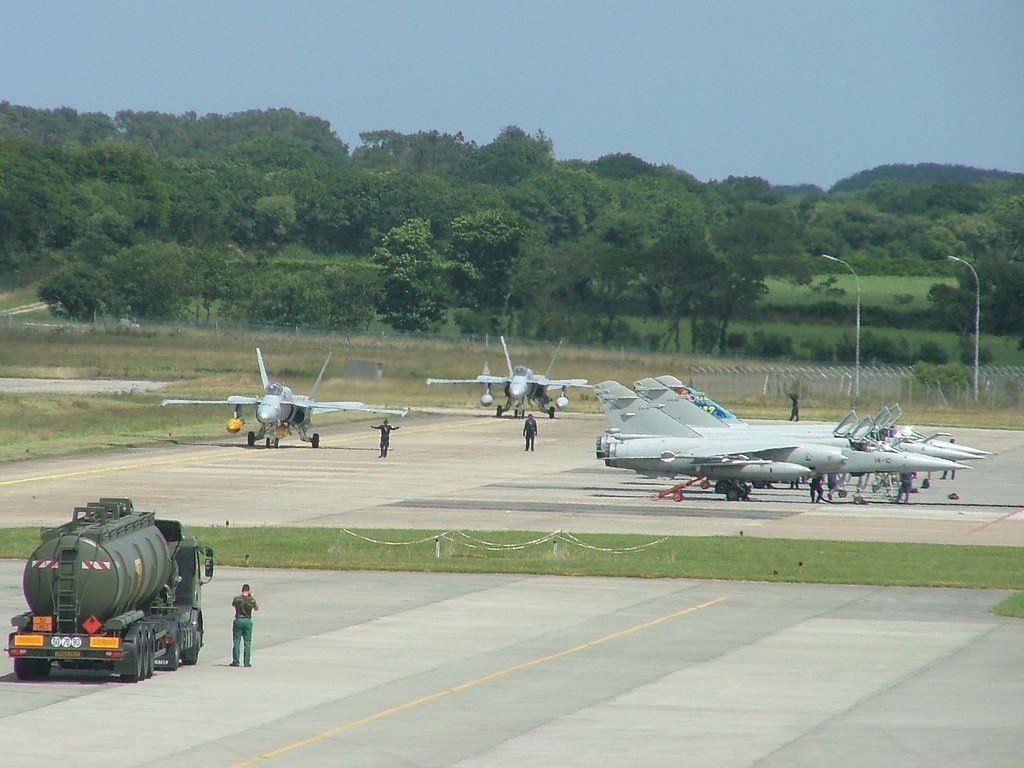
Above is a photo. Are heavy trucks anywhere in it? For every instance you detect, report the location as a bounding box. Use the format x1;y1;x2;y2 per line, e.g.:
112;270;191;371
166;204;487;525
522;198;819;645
4;494;214;683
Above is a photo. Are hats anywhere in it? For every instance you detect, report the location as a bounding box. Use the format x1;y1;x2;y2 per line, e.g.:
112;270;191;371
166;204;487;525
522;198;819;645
383;419;389;423
527;414;534;418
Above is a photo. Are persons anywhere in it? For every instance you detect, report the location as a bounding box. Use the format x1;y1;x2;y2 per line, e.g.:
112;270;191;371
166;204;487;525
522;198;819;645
791;472;837;502
523;414;538;451
789;393;800;421
229;584;258;667
370;420;399;458
940;439;956;480
894;471;917;503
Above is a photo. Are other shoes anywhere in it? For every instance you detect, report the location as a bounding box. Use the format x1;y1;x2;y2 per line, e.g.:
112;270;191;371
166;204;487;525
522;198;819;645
244;663;251;667
229;663;239;667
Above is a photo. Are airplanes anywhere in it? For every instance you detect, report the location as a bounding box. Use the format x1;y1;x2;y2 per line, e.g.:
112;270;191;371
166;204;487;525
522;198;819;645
156;347;409;448
588;365;995;504
423;334;594;419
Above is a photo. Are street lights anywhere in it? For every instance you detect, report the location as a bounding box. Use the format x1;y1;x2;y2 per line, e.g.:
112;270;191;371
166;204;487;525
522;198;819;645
821;254;862;399
947;255;980;405
